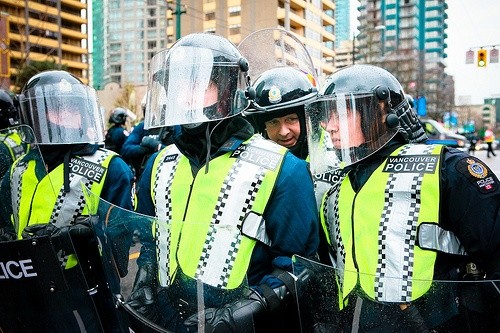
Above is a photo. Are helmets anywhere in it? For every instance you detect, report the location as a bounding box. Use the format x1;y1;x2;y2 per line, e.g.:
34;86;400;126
148;32;255;128
108;108;128;124
1;91;18;128
237;66;321;117
17;70;106;144
308;65;410;175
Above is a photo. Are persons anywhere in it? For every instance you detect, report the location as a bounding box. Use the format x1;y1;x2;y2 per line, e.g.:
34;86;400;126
306;64;500;333
468;131;479;155
105;87;182;192
0;70;134;333
0;91;30;180
236;27;348;217
485;127;497;158
127;32;319;333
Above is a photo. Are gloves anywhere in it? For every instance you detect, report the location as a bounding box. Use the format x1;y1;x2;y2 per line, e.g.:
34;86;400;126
128;246;159;317
23;223;87;238
183;286;268;333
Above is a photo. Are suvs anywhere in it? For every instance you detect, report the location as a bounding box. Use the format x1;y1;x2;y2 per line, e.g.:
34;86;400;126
419;119;469;151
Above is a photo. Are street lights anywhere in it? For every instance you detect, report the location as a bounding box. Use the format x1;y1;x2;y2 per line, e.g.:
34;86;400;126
477;50;487;68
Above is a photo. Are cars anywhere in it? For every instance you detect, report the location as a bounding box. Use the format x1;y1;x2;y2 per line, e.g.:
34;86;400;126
473;138;500;150
